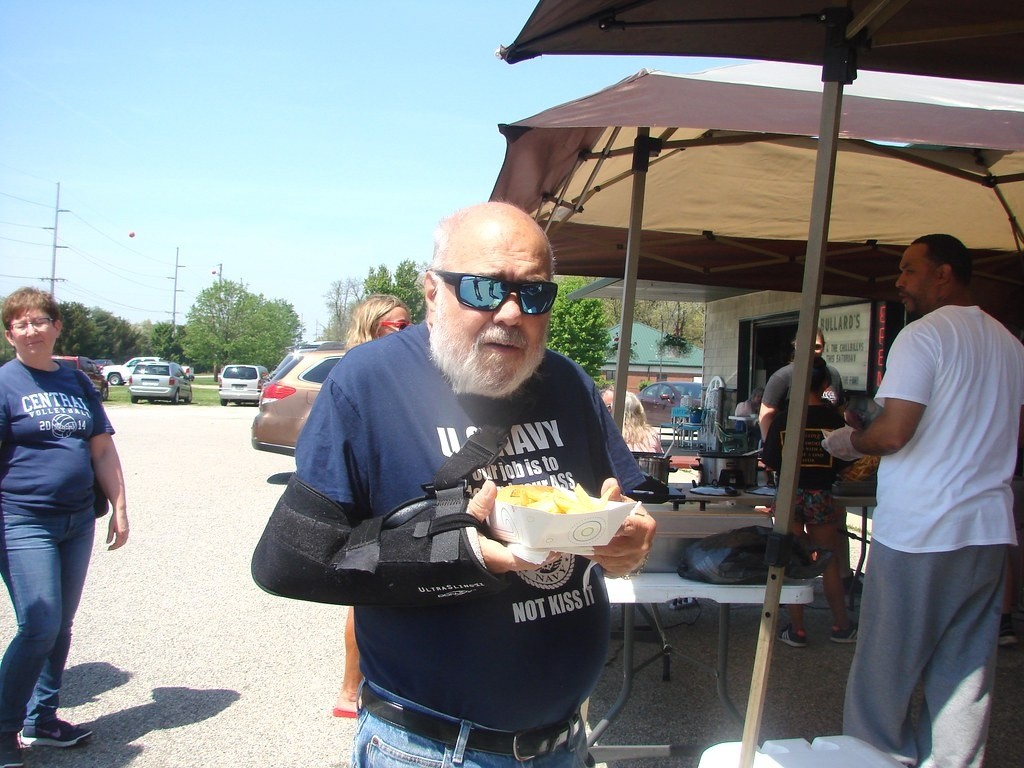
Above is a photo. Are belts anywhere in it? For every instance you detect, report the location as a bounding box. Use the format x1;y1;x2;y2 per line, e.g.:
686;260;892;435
360;680;583;761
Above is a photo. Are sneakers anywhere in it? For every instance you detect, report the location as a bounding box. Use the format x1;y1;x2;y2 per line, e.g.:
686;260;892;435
778;624;806;647
0;733;24;768
829;623;858;643
19;719;93;748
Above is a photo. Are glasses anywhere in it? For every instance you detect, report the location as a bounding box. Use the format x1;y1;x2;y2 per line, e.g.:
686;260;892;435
378;320;412;332
427;268;558;315
6;317;54;335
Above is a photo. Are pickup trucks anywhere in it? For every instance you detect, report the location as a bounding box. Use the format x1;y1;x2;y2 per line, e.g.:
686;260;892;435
100;356;195;386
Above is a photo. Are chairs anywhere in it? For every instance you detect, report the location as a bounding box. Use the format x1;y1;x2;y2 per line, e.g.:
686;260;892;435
660;406;706;449
715;422;747;453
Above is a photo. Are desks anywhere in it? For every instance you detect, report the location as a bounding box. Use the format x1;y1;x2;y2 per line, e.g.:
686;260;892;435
582;484;876;747
729;415;759;446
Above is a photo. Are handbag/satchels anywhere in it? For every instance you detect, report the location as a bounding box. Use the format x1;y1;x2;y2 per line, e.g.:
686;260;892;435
90;458;110;518
677;526;835;584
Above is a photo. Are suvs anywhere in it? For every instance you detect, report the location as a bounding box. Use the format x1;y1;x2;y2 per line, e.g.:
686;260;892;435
51;355;109;400
251;342;347;456
128;361;193;405
219;365;269;407
94;359;114;374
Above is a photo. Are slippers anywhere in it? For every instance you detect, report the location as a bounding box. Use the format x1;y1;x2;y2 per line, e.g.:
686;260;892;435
333;707;358;719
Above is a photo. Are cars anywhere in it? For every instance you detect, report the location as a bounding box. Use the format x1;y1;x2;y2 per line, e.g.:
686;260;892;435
635;381;702;428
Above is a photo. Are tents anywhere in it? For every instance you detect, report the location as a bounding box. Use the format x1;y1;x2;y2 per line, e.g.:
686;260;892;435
498;0;1023;768
480;66;1023;439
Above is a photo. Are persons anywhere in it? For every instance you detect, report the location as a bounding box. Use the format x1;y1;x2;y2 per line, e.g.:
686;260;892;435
736;386;772;470
757;323;864;599
250;203;659;768
760;359;860;648
0;286;129;768
601;387;666;475
332;292;416;716
822;231;1024;768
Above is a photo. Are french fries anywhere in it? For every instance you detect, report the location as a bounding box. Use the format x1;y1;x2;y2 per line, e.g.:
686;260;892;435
846;454;879;482
489;483;618;514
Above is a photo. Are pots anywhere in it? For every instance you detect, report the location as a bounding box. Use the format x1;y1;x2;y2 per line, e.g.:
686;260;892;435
630;452;677;486
690;446;759;486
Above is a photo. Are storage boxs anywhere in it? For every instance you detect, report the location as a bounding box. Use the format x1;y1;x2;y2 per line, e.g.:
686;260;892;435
479;484;637;549
